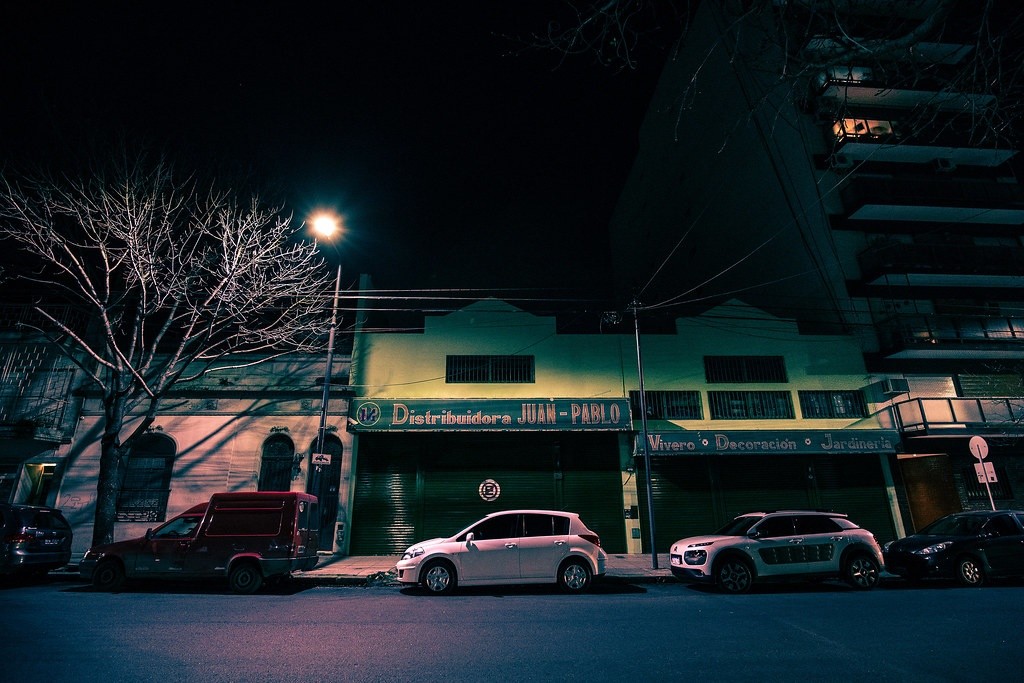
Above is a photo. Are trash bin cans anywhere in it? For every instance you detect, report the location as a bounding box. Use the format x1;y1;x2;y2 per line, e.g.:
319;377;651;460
333;521;345;553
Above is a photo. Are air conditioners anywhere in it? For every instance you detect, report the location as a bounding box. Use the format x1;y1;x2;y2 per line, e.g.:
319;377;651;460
882;377;912;395
932;156;954;170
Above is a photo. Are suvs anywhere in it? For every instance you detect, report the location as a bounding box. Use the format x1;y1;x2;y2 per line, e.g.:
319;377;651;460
78;490;320;596
669;510;886;592
1;503;73;581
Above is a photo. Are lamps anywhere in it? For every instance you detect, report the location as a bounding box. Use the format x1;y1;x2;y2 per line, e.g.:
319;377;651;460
291;452;305;476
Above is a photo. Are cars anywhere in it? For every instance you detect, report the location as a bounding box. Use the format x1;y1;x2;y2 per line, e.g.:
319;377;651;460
397;510;608;596
881;508;1024;586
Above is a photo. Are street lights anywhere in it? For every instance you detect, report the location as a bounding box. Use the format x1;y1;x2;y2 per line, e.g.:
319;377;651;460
304;208;350;494
